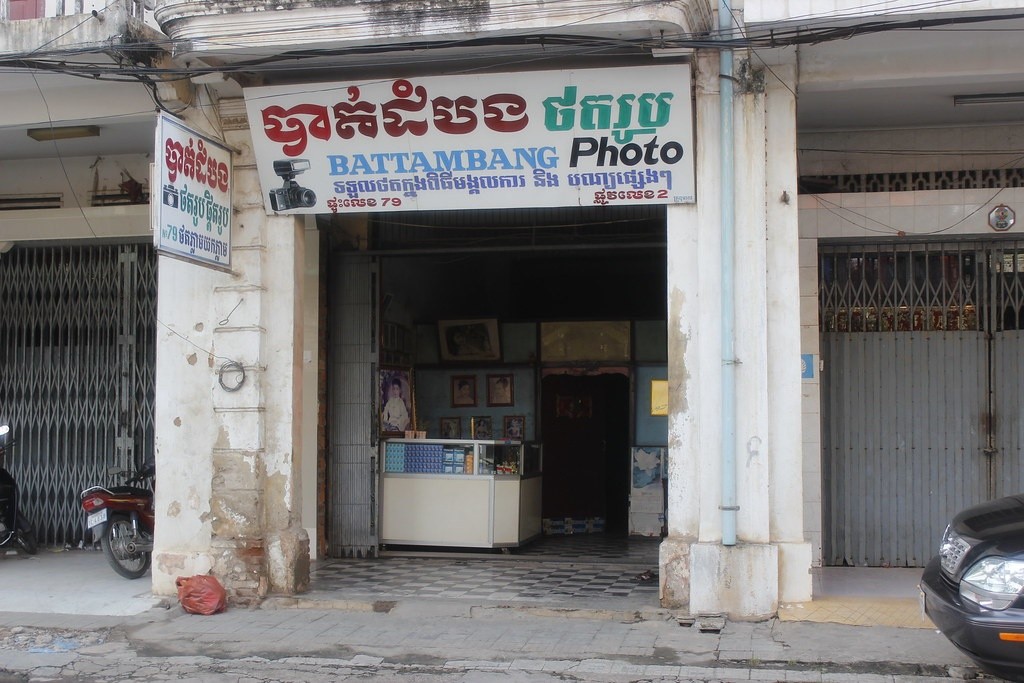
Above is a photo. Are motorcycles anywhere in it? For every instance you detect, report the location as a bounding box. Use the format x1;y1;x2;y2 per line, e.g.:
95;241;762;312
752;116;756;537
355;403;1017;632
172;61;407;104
0;423;39;555
80;455;156;580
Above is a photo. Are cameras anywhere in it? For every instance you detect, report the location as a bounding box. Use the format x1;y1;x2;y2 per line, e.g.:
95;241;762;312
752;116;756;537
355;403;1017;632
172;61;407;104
269;159;317;211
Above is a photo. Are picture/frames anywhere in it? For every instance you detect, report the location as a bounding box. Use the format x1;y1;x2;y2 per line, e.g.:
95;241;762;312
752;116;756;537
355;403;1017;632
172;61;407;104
382;321;413;365
377;362;417;438
504;416;524;440
434;315;504;363
441;416;461;439
384;292;394;312
450;375;478;407
470;415;492;440
486;374;514;407
650;378;669;416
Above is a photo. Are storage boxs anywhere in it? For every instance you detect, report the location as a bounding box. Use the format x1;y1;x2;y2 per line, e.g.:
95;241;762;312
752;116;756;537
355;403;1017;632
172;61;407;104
453;462;464;474
404;430;416;439
386;443;445;474
443;449;454;462
443;462;453;474
454;447;472;462
543;516;606;537
480;459;489;474
415;431;429;439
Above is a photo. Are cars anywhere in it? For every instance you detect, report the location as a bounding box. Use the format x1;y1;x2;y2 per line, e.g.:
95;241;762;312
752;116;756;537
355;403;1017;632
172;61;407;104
918;492;1024;683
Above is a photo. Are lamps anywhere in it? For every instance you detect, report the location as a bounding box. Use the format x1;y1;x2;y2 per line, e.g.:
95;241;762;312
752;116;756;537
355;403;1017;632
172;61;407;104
27;125;100;143
954;92;1024;107
